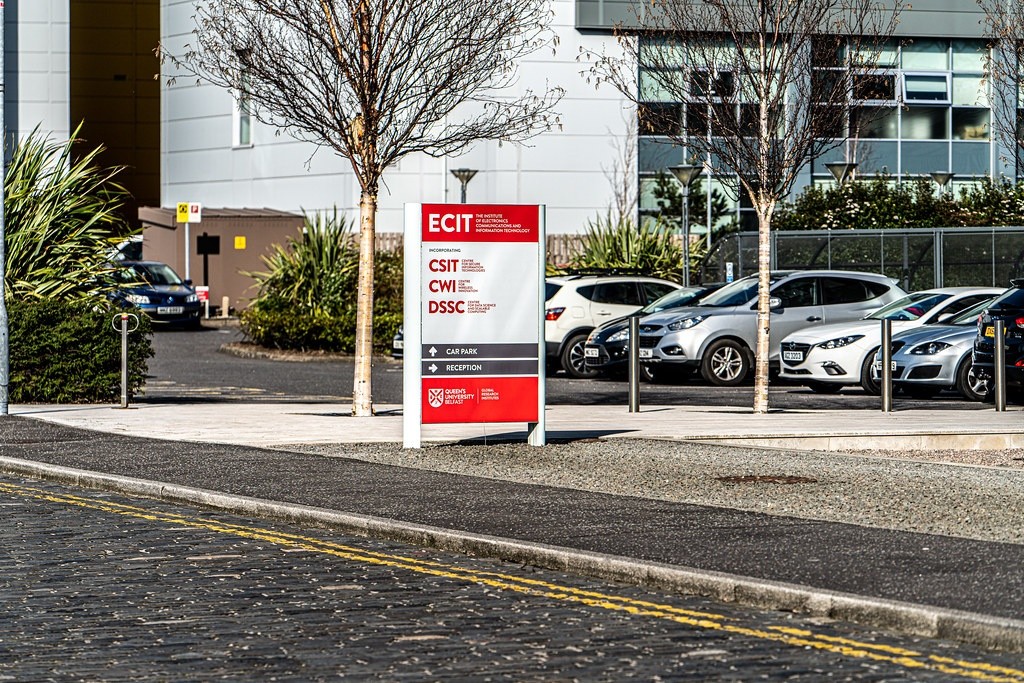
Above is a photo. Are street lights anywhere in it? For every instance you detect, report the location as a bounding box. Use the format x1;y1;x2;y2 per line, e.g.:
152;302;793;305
667;164;704;287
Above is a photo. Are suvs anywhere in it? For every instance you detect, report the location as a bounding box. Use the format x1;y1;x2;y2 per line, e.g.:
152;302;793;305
545;266;690;379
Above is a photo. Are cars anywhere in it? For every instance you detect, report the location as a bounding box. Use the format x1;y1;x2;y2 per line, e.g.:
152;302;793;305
582;267;1024;409
80;260;203;331
